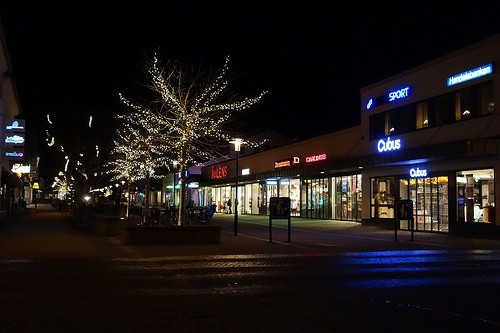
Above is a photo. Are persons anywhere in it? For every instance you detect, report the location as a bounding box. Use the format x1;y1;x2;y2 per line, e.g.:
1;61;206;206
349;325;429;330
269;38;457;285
100;190;262;216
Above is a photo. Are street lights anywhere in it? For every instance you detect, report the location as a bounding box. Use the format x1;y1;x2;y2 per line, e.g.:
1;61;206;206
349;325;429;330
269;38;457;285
230;138;247;236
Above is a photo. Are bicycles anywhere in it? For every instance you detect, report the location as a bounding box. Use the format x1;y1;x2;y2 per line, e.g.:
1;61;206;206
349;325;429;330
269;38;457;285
140;203;218;227
